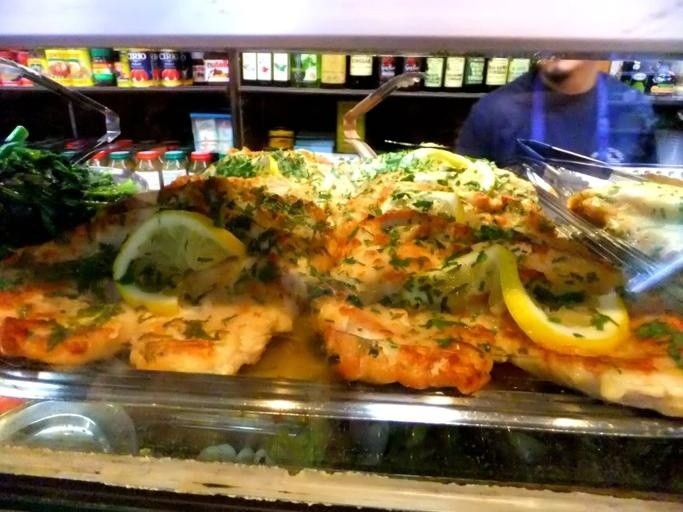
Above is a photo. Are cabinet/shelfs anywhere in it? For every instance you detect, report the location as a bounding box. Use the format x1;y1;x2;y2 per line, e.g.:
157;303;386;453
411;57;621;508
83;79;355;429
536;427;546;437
239;34;682;167
1;34;239;155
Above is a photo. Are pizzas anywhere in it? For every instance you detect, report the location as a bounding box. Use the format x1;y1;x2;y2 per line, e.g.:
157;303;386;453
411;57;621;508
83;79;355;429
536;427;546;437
0;146;683;418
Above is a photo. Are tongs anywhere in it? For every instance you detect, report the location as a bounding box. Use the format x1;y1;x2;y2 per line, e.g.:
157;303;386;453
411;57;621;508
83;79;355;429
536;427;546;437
513;131;681;313
340;71;427;159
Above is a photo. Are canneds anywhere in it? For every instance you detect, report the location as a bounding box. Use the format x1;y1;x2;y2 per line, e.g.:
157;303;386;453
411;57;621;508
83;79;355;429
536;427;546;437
1;47;194;90
61;137;214;191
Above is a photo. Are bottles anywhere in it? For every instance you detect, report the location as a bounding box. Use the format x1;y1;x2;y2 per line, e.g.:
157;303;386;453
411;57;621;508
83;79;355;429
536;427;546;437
59;134;212;190
91;48;115;88
190;50;205;84
239;49;531;92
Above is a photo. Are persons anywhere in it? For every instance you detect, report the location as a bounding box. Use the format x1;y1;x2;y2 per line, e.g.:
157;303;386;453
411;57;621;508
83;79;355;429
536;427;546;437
455;59;656;179
